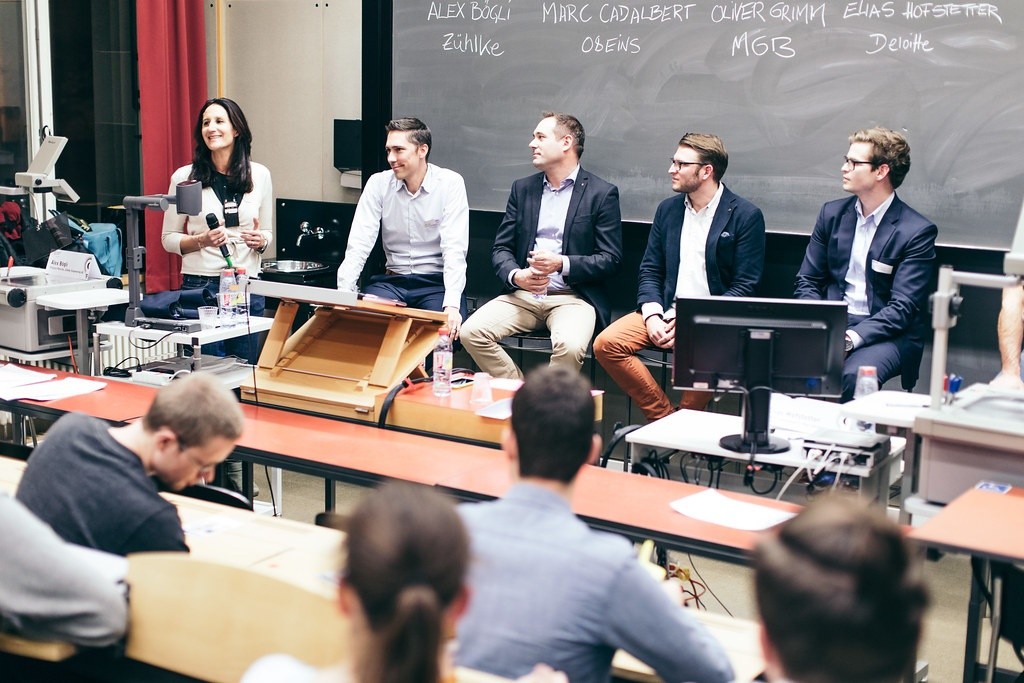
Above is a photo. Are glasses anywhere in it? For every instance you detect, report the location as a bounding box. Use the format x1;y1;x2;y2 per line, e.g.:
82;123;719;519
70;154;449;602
176;437;215;477
669;157;707;171
843;155;877;171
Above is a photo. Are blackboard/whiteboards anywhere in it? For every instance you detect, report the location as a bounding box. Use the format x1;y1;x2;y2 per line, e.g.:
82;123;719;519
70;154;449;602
362;0;1024;272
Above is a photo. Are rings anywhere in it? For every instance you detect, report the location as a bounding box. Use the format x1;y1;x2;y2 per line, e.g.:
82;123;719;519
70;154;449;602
251;242;254;245
218;239;221;242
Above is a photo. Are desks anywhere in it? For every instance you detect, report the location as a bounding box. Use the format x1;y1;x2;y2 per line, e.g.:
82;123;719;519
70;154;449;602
626;408;906;517
121;400;508;513
437;453;915;571
905;479;1024;683
0;359;160;447
0;455;334;570
92;310;283;521
250;530;769;683
102;550;522;683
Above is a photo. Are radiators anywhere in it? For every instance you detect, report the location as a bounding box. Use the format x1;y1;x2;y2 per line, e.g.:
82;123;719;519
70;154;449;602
0;333;178;428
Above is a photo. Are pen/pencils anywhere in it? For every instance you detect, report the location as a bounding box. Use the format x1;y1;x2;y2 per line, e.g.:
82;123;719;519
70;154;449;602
943;374;962;405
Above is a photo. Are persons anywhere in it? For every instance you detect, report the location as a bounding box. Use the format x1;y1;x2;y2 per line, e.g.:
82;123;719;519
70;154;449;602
593;133;766;423
1;493;134;650
241;475;569;683
448;364;735;683
459;112;622;381
17;371;246;557
748;493;930;683
793;127;938;402
161;98;273;497
988;207;1024;388
335;117;469;376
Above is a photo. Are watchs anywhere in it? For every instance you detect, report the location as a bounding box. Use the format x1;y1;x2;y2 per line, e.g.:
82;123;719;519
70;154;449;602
259;235;267;253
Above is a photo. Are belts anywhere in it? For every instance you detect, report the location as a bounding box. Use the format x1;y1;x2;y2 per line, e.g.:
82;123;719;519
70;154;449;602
547;290;577;295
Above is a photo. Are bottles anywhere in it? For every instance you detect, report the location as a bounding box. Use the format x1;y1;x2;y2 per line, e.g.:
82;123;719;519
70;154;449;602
216;293;250;325
433;328;454;396
855;366;878;397
220;268;250;325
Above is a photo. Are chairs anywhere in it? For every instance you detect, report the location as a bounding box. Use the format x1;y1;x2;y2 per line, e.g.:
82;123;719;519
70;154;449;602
425;298;674;475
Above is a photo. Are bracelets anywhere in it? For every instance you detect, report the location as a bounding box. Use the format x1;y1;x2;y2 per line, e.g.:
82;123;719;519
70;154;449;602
196;236;205;249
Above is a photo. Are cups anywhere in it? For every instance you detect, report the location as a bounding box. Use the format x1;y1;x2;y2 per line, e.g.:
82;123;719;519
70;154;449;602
198;305;218;331
852;366;878;400
470;372;492;403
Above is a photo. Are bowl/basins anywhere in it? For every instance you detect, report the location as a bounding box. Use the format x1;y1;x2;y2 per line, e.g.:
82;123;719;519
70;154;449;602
260;257;329;273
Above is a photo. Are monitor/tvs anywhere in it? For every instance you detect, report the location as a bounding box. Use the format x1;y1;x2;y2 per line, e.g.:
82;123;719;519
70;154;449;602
671;295;848;452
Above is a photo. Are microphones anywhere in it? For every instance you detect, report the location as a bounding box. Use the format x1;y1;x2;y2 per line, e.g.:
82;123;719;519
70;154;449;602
206;212;233;269
